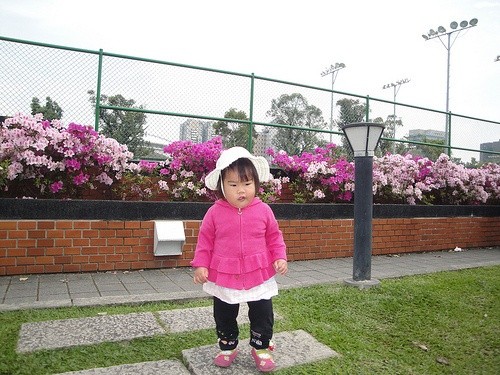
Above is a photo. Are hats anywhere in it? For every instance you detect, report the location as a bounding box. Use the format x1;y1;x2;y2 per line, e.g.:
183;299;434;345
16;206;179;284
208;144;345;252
205;147;270;198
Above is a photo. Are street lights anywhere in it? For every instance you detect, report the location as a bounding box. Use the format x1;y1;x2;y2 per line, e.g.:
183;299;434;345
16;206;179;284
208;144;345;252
382;78;410;153
422;18;478;159
341;123;385;291
320;63;345;144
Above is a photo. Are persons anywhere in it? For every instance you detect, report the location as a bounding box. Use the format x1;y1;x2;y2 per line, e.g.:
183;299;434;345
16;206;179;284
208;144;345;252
190;146;286;372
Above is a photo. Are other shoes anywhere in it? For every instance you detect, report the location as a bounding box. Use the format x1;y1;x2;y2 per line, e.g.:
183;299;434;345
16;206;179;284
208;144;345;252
251;348;276;372
213;346;238;367
